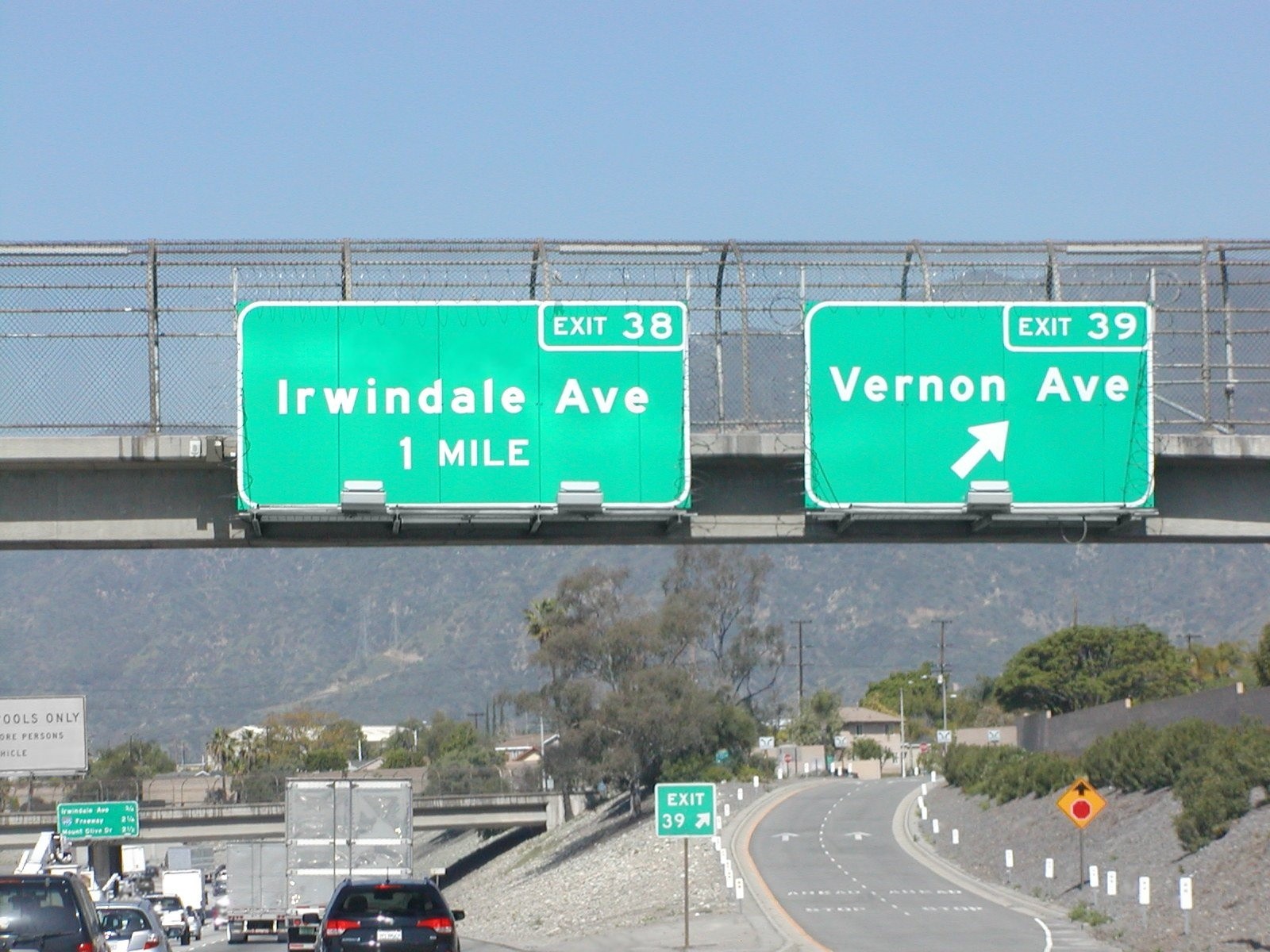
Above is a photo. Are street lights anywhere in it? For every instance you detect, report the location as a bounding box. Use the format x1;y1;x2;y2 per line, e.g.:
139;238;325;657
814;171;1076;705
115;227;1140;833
900;679;915;778
920;673;949;758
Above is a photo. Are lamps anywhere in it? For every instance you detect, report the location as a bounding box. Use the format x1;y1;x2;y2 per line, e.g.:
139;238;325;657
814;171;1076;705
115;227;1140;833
341;480;387;513
556;482;602;513
967;482;1011;512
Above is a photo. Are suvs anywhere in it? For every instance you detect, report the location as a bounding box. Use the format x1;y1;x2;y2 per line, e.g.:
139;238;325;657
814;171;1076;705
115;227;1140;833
143;891;191;946
304;876;467;951
0;872;113;952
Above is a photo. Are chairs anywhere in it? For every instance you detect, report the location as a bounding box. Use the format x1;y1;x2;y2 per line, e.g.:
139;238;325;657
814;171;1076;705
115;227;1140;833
349;896;368;914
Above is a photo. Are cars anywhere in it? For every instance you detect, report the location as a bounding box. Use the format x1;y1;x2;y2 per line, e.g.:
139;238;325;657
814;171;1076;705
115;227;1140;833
94;901;172;952
186;907;204;939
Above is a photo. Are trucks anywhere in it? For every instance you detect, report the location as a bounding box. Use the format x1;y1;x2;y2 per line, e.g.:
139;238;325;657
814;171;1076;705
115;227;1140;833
162;868;208;926
222;778;415;944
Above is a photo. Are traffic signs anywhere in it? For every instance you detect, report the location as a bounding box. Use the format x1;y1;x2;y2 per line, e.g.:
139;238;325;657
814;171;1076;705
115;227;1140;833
805;302;1156;510
654;782;716;836
